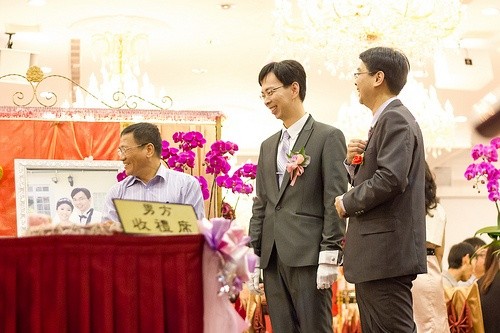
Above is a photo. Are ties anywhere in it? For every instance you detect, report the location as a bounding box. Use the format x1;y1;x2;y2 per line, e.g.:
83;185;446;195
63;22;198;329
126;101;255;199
277;131;290;189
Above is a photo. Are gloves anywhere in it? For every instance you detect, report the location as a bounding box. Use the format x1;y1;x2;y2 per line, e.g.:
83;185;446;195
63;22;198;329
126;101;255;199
317;264;338;289
248;266;262;296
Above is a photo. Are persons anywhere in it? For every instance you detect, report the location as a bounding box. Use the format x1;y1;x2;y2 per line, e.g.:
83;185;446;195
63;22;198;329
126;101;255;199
102;123;207;223
56;197;75;225
334;46;428;333
441;242;476;288
71;187;103;226
410;160;451;333
457;237;488;287
246;59;348;333
478;240;500;333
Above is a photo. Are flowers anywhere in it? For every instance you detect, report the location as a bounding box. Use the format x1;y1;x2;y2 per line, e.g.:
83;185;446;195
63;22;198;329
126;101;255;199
117;131;258;303
284;147;312;186
350;154;364;166
464;137;500;249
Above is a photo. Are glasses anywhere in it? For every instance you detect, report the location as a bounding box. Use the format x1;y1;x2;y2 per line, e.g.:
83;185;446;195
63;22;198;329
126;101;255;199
116;142;148;157
353;71;374;79
259;86;285;100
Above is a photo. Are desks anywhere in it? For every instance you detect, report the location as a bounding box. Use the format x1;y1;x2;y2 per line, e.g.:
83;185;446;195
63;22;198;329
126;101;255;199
0;233;207;333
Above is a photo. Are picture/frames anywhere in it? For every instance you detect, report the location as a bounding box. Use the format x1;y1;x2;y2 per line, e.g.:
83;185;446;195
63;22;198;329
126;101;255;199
14;158;126;237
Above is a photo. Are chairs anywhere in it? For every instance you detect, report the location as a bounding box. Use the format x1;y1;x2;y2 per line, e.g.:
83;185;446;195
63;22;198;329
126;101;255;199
447;282;485;333
